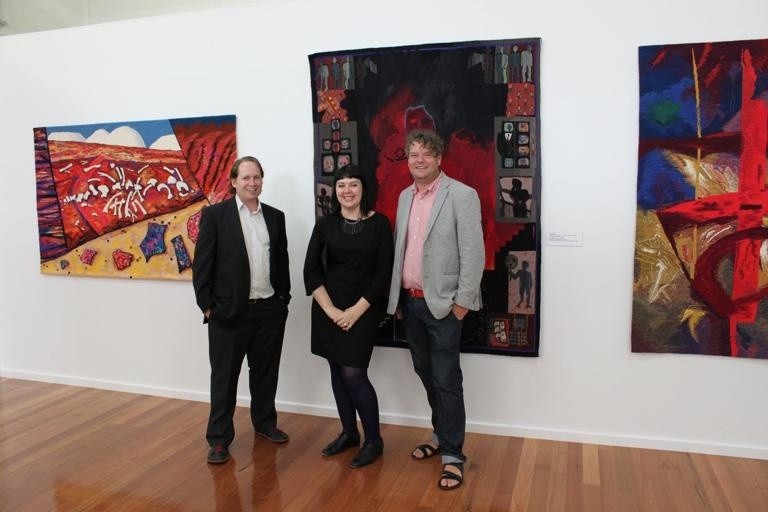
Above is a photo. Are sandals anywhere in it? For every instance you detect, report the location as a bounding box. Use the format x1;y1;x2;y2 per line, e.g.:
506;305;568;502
439;459;463;490
411;442;441;458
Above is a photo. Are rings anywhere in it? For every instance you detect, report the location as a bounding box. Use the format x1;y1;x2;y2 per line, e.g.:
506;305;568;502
344;323;348;325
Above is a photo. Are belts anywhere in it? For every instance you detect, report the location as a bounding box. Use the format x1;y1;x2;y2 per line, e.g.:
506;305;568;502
245;298;276;306
405;288;425;297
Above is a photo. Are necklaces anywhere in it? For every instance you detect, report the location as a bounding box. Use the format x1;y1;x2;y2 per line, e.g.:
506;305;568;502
341;213;363;234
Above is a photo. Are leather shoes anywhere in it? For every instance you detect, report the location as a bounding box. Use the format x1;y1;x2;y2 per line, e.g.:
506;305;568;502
350;437;384;468
255;425;289;443
323;431;361;455
206;441;229;463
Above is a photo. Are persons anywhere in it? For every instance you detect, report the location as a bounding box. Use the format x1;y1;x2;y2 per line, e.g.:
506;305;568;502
192;156;292;463
386;130;486;490
303;163;394;468
